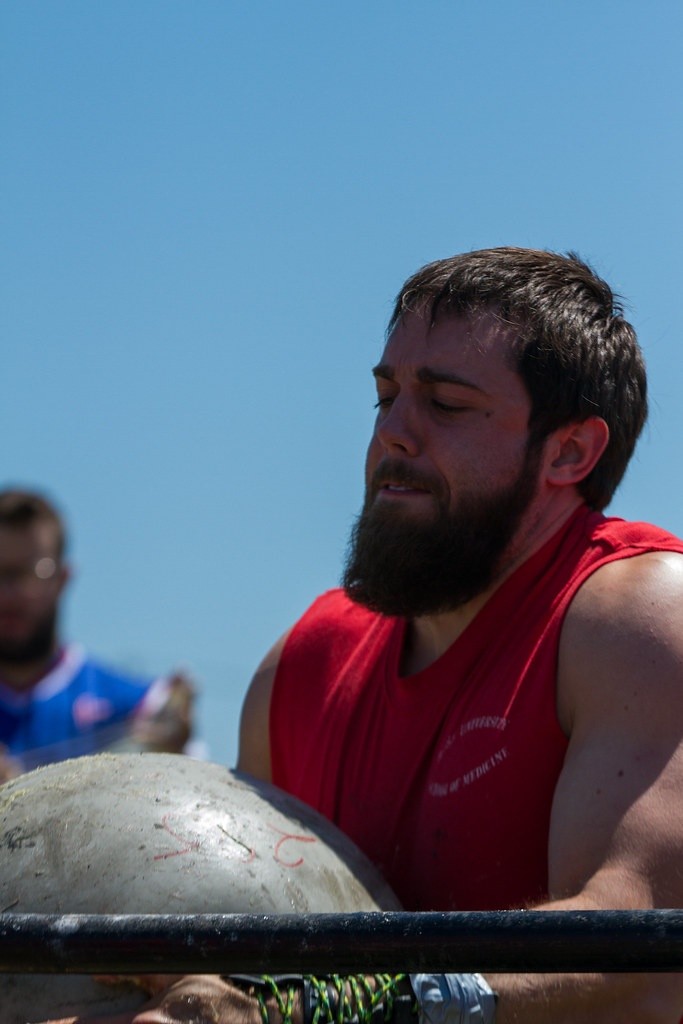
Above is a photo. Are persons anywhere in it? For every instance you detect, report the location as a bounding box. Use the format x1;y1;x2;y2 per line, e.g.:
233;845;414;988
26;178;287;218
83;243;683;1023
0;485;209;787
